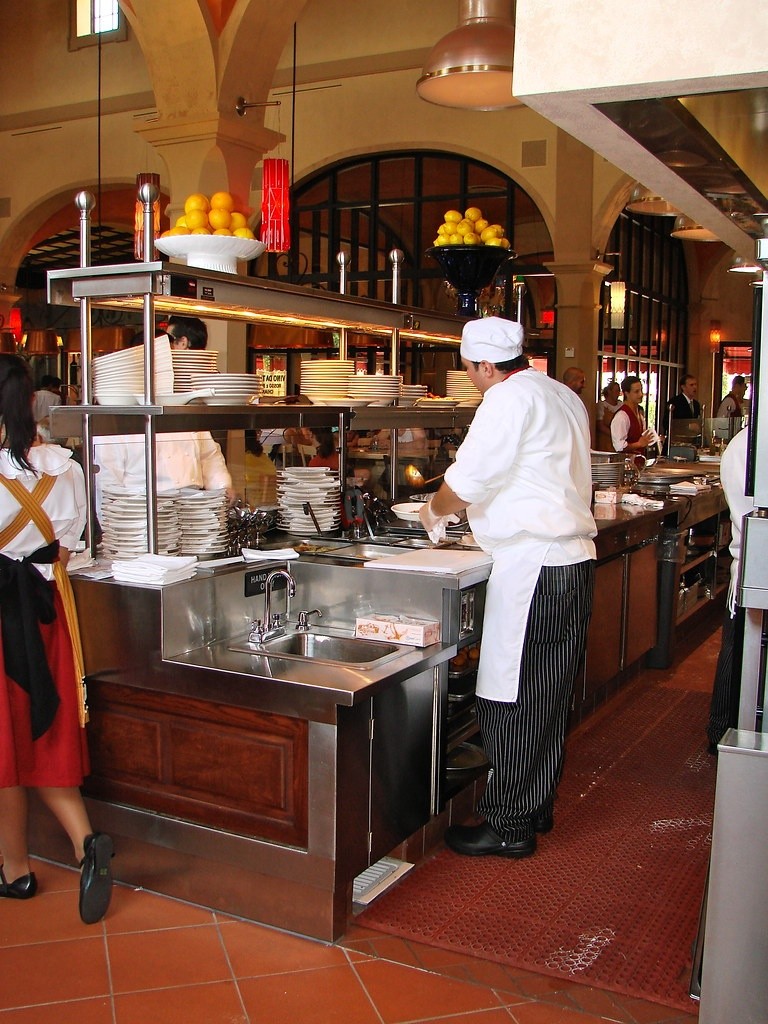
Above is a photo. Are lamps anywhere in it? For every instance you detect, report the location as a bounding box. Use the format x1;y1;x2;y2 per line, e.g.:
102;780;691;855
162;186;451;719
234;95;289;252
416;1;526;111
596;249;626;329
133;141;161;261
699;294;721;354
625;182;764;287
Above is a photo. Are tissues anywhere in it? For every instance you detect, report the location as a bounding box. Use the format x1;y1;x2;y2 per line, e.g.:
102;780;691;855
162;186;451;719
595;486;629;504
355;612;441;649
593;503;625;520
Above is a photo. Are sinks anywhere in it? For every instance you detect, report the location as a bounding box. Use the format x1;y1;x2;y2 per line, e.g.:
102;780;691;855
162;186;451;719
262;633;416;671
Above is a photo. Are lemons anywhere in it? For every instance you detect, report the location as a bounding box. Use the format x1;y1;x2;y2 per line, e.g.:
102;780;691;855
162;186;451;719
158;191;256;240
432;207;512;248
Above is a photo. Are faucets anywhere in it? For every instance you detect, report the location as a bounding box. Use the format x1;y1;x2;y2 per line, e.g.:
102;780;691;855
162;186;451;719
263;568;296;632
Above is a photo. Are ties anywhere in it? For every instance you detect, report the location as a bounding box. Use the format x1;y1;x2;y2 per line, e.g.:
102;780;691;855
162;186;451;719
689;401;693;415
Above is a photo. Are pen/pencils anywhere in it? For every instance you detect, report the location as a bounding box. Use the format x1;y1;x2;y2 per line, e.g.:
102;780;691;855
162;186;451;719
306;502;322;535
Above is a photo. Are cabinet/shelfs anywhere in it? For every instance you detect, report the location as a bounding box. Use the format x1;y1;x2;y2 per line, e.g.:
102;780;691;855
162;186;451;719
44;183;541;560
670;486;732;627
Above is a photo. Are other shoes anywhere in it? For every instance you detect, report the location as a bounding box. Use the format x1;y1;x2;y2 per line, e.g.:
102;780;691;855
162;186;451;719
709;742;718;757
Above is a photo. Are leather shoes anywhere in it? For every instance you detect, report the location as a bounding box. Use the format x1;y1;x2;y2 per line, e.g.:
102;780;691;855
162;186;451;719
535;820;553;832
0;864;37;898
78;832;114;924
445;821;536;858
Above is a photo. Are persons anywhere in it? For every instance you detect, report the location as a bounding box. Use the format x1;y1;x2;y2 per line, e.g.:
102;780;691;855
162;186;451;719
596;382;623;452
706;425;746;754
610;377;655;470
661;375;702;457
717;376;747;445
419;318;597;858
0;358;116;923
562;366;586;394
0;315;430;532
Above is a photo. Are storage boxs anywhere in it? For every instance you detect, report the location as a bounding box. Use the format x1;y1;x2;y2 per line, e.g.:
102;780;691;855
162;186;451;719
355;613;441;648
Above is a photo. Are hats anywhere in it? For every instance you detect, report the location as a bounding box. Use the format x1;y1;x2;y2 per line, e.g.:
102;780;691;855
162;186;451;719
460;316;523;363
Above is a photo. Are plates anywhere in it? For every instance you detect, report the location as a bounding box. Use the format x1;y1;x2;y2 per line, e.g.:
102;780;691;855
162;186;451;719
592;460;627;491
91;334;489;563
